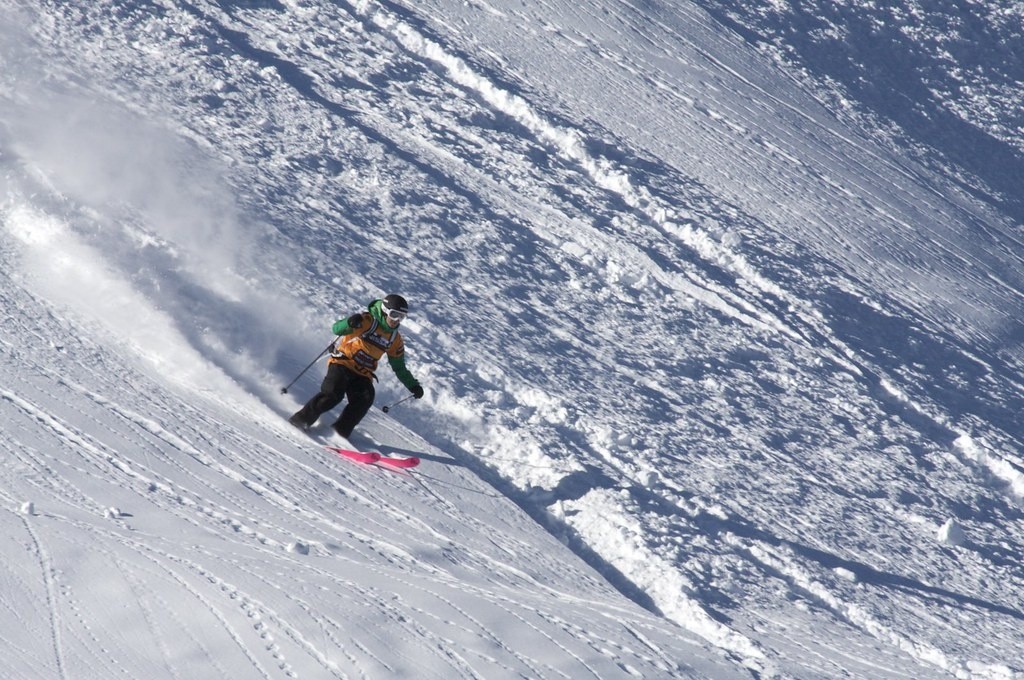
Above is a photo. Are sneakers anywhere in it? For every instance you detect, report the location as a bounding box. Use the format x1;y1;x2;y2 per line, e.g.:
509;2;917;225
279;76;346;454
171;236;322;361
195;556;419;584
289;413;312;436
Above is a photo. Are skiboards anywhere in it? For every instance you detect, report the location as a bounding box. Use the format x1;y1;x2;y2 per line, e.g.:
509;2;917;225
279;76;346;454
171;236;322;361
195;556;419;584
329;448;420;468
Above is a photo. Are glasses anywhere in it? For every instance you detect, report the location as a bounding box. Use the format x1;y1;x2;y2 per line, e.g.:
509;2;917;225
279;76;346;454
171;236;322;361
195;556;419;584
381;303;407;322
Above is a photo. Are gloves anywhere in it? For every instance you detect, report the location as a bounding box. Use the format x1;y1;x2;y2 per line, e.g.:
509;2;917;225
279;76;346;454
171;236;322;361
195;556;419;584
412;384;423;398
348;313;363;328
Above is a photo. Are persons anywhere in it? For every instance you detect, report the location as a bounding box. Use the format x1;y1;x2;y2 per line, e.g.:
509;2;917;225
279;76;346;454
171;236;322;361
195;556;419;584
288;293;425;439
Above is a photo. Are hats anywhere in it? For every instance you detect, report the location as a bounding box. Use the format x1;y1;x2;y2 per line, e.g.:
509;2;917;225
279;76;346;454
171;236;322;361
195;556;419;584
383;294;408;313
